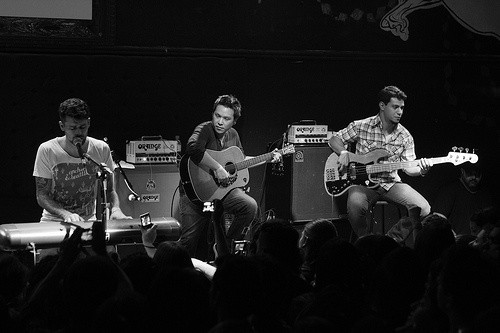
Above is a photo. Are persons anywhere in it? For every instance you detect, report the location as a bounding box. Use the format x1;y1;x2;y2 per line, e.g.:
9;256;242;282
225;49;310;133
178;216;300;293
328;86;432;248
33;98;134;263
0;199;500;333
180;95;283;263
434;163;500;250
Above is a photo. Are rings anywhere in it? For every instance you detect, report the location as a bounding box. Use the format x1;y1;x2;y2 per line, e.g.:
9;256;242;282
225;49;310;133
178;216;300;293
424;168;427;170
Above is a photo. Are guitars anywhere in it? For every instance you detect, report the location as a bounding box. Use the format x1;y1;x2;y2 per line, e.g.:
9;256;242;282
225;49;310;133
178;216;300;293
324;146;478;197
179;143;296;206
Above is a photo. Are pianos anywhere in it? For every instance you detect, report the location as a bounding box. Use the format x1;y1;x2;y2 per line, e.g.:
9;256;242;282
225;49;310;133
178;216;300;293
0;216;182;251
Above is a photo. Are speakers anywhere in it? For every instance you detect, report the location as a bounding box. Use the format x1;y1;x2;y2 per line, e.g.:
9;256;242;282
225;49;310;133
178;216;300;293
285;143;348;223
114;163;181;219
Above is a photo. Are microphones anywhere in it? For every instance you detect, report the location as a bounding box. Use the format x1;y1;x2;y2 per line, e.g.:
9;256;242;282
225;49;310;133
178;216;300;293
73;138;84;159
128;194;140;201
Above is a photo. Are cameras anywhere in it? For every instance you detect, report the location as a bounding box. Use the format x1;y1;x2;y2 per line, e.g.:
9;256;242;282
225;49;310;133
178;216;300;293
234;240;248;251
140;212;152;229
202;201;214;212
